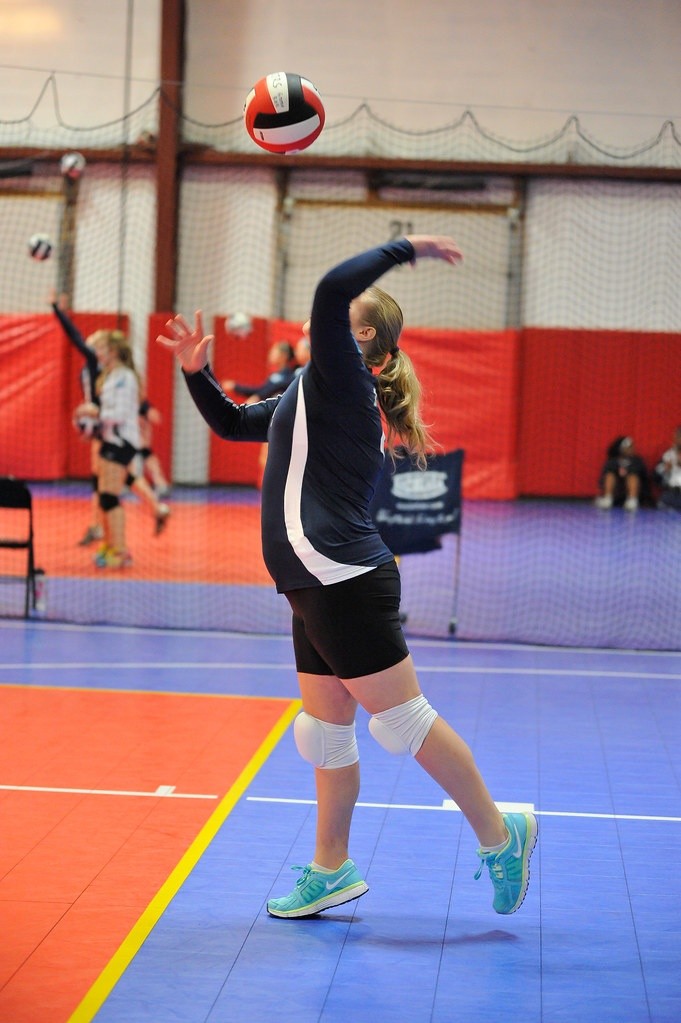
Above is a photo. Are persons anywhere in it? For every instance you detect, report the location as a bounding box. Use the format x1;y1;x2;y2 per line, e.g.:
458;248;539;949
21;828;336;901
593;436;648;510
239;338;312;408
221;341;294;491
156;235;542;920
654;425;681;514
49;288;172;569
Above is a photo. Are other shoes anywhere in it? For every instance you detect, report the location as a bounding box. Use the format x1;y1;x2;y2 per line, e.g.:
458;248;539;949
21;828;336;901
154;487;169;499
80;525;104;544
118;487;140;507
624;498;638;512
154;503;169;535
95;545;130;568
598;496;613;509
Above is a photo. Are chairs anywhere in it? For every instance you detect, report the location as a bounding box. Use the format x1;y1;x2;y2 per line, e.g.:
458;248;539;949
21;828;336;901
367;445;465;638
0;476;37;618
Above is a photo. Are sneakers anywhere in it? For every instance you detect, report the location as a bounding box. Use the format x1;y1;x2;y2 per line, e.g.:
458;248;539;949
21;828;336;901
267;859;370;918
473;812;538;916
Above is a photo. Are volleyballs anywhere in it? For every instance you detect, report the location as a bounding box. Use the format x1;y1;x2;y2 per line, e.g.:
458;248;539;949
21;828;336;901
74;400;103;437
61;154;87;178
241;70;327;157
225;309;252;339
29;233;53;261
